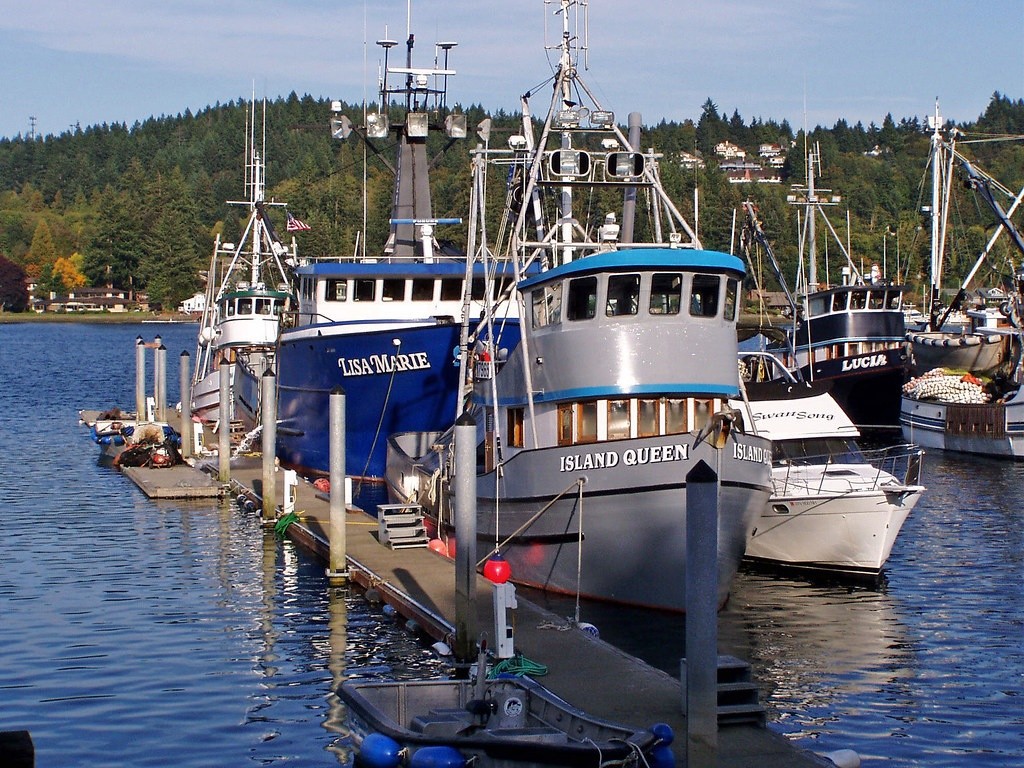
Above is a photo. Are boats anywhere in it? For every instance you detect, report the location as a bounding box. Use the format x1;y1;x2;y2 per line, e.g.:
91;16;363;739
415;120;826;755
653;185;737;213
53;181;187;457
715;85;1024;584
338;675;661;768
153;0;769;619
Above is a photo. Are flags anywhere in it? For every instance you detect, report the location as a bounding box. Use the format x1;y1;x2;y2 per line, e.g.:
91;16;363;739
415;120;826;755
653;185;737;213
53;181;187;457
287;212;310;230
